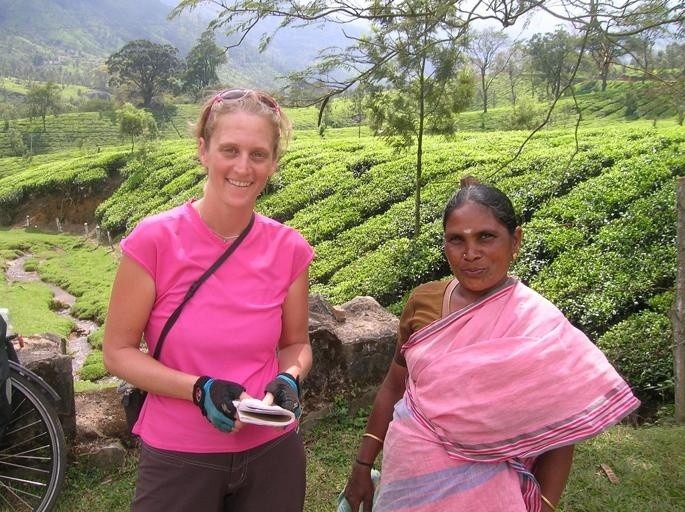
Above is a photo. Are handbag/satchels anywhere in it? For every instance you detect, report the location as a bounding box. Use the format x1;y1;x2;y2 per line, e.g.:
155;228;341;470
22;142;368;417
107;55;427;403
115;378;146;437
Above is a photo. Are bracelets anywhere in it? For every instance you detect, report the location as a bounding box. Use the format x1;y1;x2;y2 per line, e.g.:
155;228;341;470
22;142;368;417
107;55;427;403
540;493;557;512
355;459;374;467
361;433;384;444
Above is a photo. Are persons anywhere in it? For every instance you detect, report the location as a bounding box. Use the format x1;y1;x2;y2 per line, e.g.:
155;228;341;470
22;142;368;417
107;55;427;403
343;177;641;511
102;88;313;512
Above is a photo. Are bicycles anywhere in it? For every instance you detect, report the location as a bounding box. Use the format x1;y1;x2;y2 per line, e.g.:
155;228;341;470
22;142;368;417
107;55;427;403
1;334;66;512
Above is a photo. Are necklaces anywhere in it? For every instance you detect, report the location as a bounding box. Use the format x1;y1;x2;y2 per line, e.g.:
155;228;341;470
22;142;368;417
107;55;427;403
197;199;241;243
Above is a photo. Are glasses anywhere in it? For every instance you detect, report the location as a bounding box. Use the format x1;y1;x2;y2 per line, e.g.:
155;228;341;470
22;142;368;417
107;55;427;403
201;87;282;134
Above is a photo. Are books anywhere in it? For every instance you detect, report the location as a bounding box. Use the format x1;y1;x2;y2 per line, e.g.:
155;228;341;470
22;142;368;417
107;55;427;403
231;398;296;427
